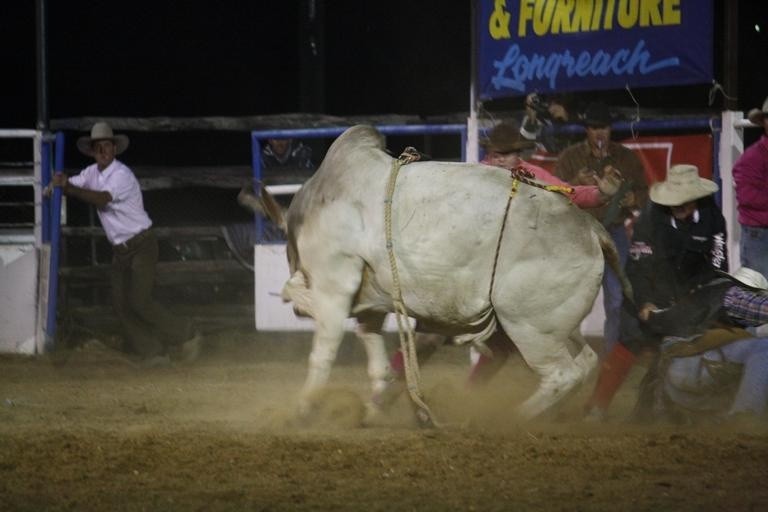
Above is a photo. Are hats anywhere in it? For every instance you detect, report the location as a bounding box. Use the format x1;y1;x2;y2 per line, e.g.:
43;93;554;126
649;164;719;207
76;122;130;157
574;101;628;128
749;99;768;123
712;266;768;291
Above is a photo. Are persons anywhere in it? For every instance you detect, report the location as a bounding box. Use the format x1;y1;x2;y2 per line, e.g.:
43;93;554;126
37;123;203;366
382;119;624;401
522;88;766;425
259;134;315;176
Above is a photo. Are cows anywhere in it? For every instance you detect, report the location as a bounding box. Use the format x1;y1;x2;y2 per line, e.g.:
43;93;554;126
238;123;665;435
